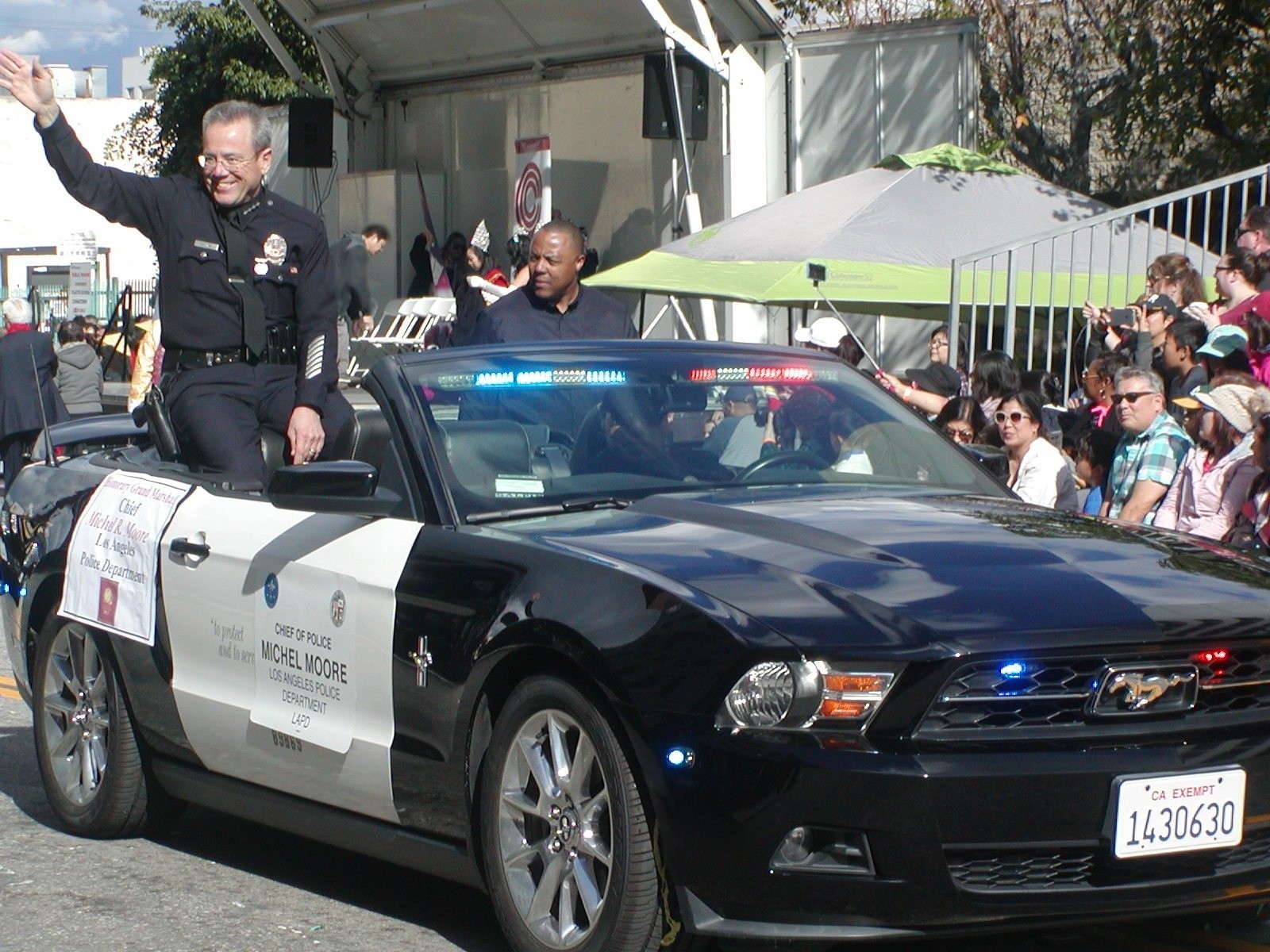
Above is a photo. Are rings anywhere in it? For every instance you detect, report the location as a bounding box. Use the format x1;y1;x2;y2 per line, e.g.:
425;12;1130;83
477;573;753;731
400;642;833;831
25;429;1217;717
310;448;315;454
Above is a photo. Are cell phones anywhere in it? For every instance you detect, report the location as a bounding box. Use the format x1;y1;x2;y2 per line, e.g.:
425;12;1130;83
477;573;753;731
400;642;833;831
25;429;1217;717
1106;308;1135;325
806;263;828;281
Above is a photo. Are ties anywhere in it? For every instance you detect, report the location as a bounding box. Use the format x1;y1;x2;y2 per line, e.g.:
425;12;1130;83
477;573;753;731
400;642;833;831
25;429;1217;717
224;206;267;357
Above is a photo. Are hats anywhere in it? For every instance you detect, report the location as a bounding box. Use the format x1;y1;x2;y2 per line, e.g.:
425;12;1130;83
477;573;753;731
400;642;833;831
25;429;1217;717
1171;385;1209;408
1129;293;1179;322
1195;324;1249;357
905;366;961;396
794;317;847;348
725;385;758;404
1194;384;1257;433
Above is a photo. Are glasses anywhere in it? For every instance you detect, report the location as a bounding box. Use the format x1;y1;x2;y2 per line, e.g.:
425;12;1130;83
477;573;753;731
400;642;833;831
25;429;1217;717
1082;370;1102;383
1149;275;1172;287
1238;227;1256;240
995;411;1033;425
945;429;974;442
198;146;268;173
1216;266;1239;275
1111;392;1158;404
928;339;948;349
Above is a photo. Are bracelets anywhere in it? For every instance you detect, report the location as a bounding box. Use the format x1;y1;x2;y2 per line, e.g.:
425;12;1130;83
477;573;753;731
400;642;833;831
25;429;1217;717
903;387;913;403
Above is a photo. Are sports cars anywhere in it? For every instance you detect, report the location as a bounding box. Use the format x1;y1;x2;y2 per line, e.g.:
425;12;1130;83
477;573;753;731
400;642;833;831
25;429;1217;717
0;339;1270;952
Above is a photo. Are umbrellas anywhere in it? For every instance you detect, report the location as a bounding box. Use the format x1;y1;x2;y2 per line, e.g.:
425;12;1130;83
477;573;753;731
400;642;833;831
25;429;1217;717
582;142;1224;336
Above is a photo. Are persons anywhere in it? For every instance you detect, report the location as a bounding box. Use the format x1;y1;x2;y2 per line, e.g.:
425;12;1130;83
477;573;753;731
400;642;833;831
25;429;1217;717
329;224;391;385
703;411;725;440
466;243;509;308
700;385;766;469
1153;384;1264;544
769;205;1270;572
51;320;104;456
568;387;673;478
407;228;466;339
1;315;165;413
1;297;71;497
0;47;357;492
993;388;1078;512
445;218;639;464
1098;365;1196;525
577;226;600;281
466;228;531;298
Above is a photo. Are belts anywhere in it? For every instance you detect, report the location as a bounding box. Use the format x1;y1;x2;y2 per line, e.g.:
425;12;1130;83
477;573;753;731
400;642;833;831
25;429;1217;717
193;349;268;367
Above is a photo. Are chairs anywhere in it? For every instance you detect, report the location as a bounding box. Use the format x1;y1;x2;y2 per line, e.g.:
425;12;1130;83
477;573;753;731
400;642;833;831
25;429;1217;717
338;295;457;388
435;418;532;494
260;408;399;499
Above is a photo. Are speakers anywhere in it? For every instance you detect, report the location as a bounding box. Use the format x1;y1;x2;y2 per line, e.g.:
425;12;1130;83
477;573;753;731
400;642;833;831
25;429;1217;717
288;97;335;168
642;53;709;142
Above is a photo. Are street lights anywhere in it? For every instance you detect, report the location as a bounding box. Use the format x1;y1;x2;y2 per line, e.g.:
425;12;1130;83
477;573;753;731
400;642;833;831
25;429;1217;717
97;247;111;292
